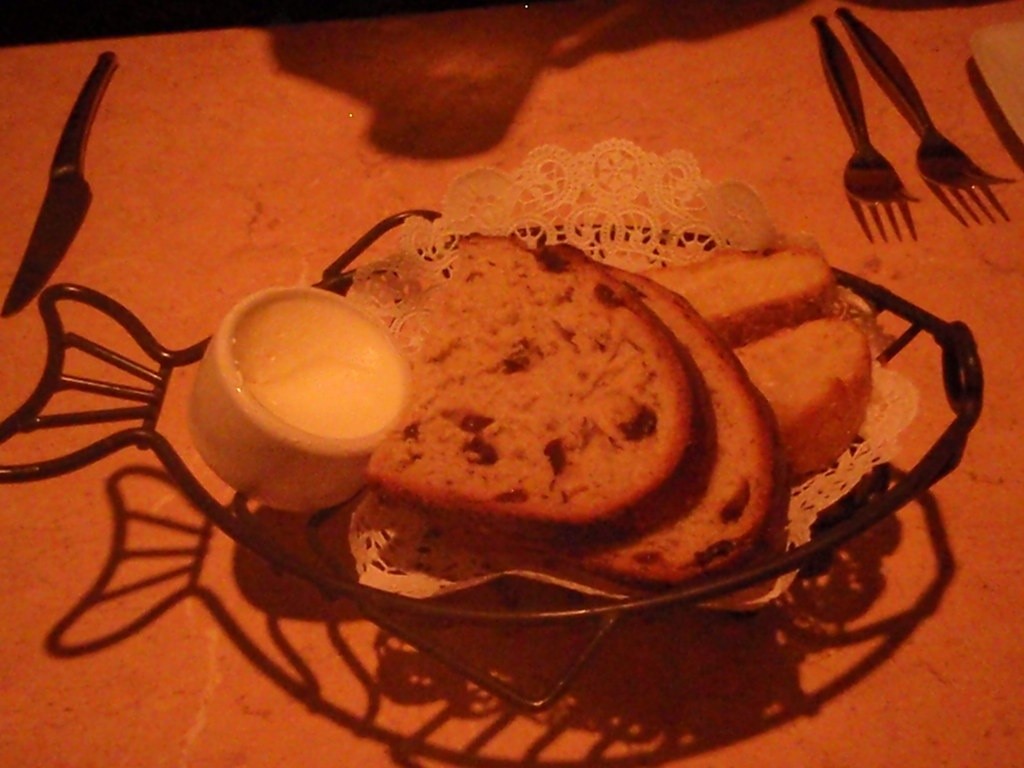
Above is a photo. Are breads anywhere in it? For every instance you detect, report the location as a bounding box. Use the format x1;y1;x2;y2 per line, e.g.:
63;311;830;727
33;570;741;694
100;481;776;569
729;318;870;479
636;233;835;346
345;232;782;591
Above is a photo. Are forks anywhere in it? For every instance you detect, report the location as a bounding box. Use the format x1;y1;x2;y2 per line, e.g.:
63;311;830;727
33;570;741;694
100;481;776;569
835;6;1019;189
810;13;922;206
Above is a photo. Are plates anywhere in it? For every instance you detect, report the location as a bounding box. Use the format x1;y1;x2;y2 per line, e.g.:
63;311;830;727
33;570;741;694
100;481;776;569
970;20;1024;154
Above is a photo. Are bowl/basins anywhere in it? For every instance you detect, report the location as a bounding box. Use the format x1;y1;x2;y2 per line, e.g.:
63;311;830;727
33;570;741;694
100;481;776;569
187;285;411;511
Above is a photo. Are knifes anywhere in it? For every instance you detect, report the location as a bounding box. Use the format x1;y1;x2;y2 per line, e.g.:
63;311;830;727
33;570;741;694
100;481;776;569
0;50;119;317
